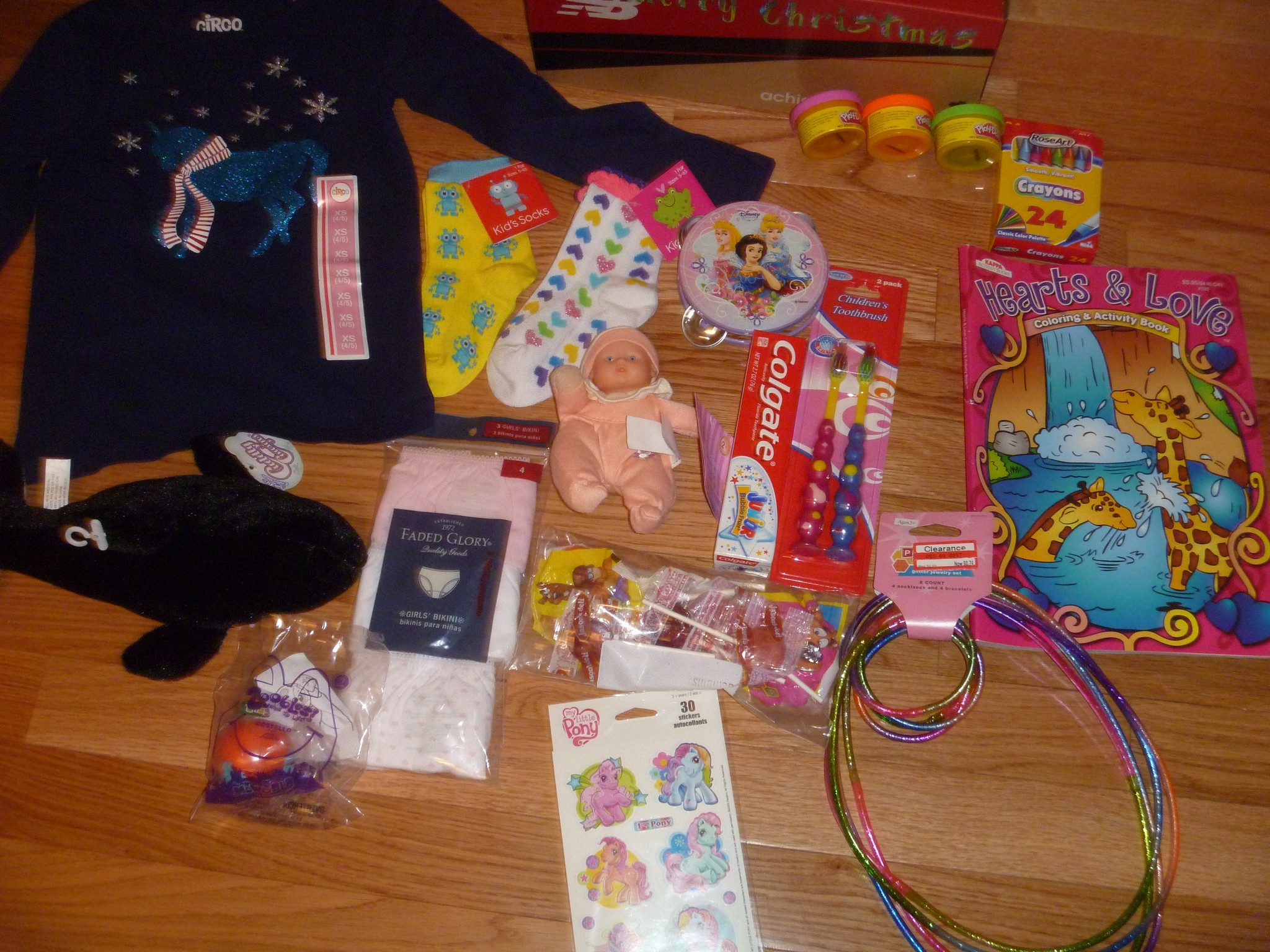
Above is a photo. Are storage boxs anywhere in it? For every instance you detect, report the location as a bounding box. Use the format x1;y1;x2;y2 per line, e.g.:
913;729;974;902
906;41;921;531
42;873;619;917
525;0;1016;74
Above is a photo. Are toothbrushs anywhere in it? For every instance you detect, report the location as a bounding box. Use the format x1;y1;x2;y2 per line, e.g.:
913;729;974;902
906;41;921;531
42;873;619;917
825;340;881;565
791;339;850;561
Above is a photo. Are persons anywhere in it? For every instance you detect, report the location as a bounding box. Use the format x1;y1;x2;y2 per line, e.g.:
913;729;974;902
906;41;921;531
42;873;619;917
548;325;700;534
